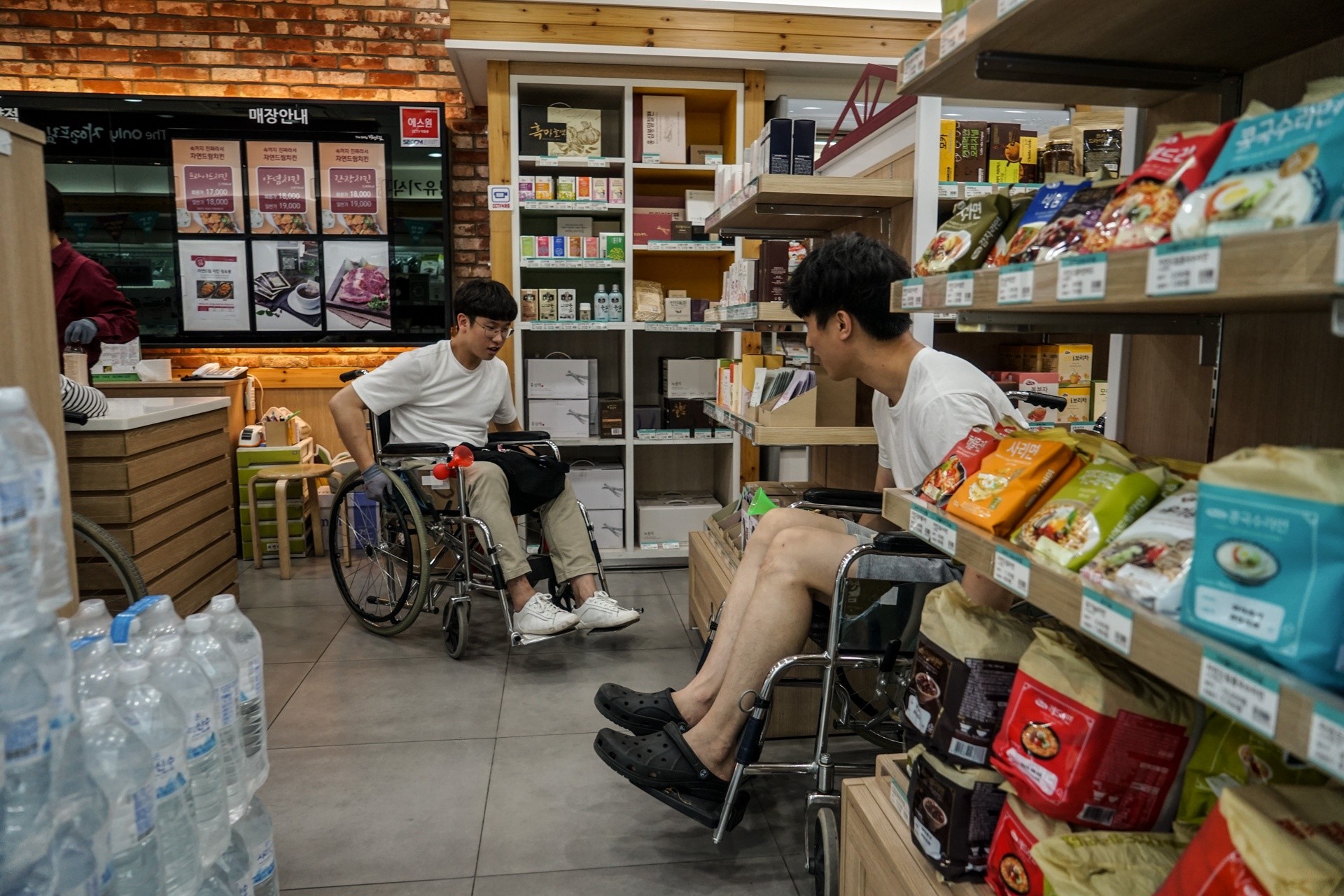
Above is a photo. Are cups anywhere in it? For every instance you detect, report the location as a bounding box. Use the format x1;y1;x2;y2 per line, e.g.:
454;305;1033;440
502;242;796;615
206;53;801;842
296;283;322;309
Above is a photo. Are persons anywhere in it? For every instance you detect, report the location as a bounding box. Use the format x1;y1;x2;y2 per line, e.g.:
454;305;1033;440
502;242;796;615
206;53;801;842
327;278;640;634
593;233;1028;828
45;179;140;418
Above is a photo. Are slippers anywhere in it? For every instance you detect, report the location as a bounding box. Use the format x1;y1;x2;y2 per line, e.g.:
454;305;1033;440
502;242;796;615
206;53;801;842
594;720;740;805
595;682;692;735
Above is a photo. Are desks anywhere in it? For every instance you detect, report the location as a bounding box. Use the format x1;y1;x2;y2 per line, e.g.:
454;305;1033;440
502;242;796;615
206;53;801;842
91;360;256;548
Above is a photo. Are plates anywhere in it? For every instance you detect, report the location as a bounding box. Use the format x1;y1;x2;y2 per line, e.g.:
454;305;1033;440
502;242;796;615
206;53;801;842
321;209;335;229
216;283;232;297
288;290;322;315
199;282;215;297
250;208;264;228
177;207;192;227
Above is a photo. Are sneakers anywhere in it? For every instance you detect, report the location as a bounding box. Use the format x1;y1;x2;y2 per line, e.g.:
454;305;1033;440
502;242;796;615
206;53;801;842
571;591;640;629
511;591;580;635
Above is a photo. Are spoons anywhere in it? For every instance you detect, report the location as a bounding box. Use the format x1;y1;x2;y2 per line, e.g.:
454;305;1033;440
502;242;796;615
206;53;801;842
194;212;210;233
337;214;354;234
265;213;281;233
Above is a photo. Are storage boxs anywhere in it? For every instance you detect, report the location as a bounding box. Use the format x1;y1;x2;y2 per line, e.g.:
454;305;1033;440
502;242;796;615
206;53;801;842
516;176;625;258
527;351;737;441
583;508;625;551
714;117;816;308
561;457;628;509
639;94;687;165
689;144;727;164
637;487;723;553
317;478;389;562
518;103;621;158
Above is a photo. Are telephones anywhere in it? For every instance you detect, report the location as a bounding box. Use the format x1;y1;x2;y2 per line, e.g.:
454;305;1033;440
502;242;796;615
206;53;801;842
192;362;249;380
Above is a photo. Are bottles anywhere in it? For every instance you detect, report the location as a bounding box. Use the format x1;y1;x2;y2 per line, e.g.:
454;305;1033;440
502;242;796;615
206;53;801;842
608;284;623;322
63;341;89;386
0;387;75;638
594;284;608;321
1;593;280;896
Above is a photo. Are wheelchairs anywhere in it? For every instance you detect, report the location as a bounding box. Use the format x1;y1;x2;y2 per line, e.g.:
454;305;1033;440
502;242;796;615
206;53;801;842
61;406;150;614
630;392;1107;896
327;368;645;660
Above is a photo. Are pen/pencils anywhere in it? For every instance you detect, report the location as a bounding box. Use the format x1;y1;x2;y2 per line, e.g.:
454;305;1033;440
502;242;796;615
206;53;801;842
265;410;301;422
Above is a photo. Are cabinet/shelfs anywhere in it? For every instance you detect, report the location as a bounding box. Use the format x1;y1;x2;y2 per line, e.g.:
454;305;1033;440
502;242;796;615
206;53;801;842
471;37;778;583
684;171;921;643
838;0;1344;896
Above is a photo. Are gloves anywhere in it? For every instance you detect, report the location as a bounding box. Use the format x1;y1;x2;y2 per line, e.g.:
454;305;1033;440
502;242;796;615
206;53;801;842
64;318;98;345
360;462;392;505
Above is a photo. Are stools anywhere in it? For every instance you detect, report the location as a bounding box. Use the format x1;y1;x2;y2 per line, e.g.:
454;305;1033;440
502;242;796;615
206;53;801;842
247;461;351;586
